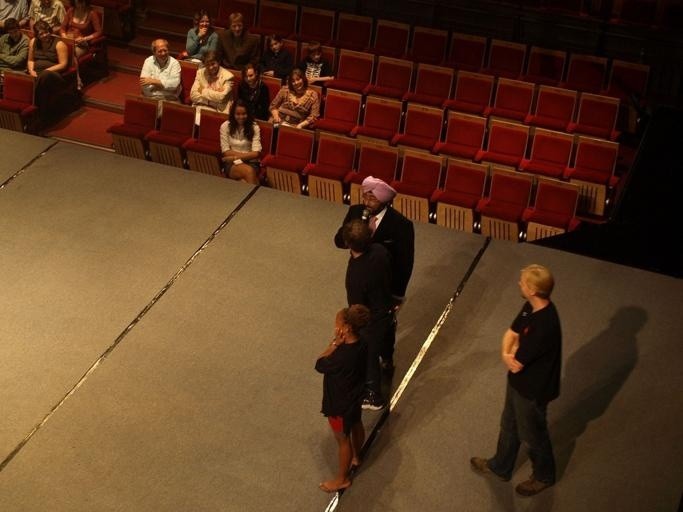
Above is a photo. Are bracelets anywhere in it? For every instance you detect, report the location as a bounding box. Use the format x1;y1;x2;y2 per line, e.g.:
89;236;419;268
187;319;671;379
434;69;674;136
331;341;340;347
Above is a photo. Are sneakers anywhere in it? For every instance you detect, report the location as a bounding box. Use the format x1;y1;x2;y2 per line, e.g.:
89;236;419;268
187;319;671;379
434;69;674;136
361;397;382;410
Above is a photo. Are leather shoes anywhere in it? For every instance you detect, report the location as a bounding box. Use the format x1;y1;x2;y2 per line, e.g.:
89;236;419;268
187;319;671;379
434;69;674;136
470;457;512;482
515;476;549;496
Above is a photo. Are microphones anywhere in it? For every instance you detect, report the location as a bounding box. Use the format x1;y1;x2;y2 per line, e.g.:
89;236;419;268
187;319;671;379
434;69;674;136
361;210;369;224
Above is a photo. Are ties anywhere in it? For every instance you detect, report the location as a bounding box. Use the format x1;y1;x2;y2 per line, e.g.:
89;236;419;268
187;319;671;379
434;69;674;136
368;217;377;230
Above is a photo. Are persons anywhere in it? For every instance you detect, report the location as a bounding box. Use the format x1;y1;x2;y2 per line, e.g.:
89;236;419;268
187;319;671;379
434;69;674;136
0;0;116;95
341;219;393;410
313;302;372;494
180;10;217;62
467;261;562;497
297;42;338;85
230;63;271;123
132;34;183;102
188;53;234;113
332;171;414;412
267;67;322;131
213;99;263;187
258;32;292;77
217;12;260;69
609;132;641;211
